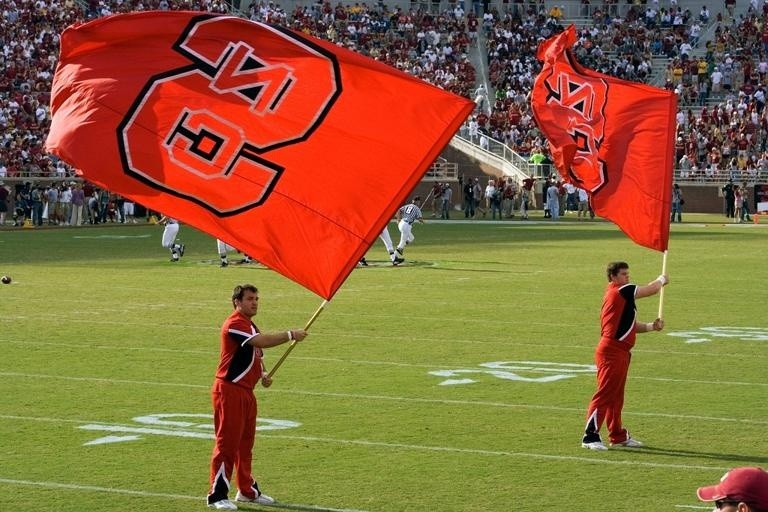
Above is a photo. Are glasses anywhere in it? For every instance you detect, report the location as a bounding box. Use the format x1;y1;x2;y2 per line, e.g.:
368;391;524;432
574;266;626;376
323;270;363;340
715;498;744;509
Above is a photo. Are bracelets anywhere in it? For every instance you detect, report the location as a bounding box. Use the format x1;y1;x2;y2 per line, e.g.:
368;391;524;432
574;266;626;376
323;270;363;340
646;322;654;332
262;372;268;376
287;330;295;341
655;275;666;285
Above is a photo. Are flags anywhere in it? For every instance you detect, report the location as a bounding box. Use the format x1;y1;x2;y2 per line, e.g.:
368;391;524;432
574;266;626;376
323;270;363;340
42;8;478;302
528;20;681;255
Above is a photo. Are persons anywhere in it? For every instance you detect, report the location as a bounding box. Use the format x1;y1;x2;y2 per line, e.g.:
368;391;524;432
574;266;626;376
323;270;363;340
161;0;464;103
242;254;259;265
430;180;453;220
217;237;241;267
156;212;185;263
460;0;768;222
358;226;405;268
204;284;309;510
394;194;424;255
579;259;671;451
1;0;161;225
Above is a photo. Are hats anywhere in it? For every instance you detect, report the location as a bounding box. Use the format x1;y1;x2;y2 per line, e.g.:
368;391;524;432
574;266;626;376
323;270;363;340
412;195;422;201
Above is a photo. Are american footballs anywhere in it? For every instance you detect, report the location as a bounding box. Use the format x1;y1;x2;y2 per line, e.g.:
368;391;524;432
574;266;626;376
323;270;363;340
1;276;10;283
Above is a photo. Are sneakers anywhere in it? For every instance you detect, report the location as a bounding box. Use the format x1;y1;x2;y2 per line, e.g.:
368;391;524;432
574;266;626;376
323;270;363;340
171;258;179;262
464;211;487;219
492;214;529;220
726;215;753;223
12;221;82;227
220;256;227;267
582;439;608;452
178;244;185;257
235;489;275;505
89;219;138;224
207;497;237;511
609;436;642;447
429;213;449;220
576;217;589;221
358;258;368;267
544;214;564;221
396;247;403;256
671;221;683;224
393;258;404;265
245;256;252;263
